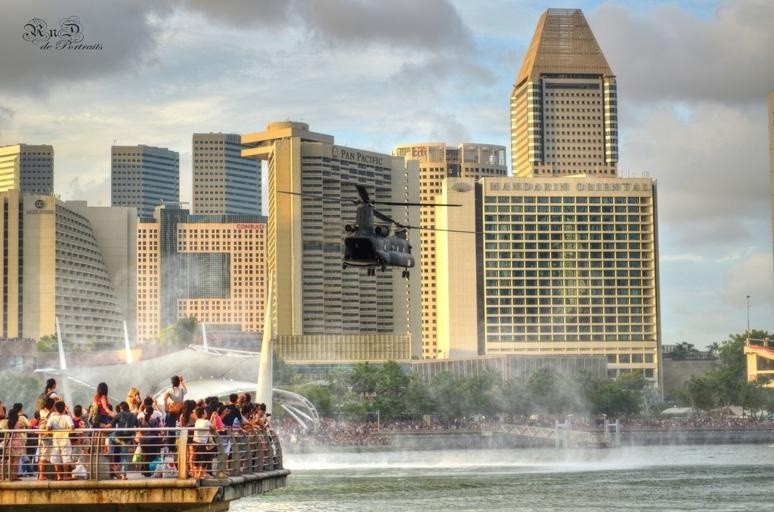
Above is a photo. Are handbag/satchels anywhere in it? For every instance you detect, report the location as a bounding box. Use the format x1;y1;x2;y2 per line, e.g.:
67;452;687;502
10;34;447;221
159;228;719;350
88;403;99;425
39;418;50;440
169;401;184;416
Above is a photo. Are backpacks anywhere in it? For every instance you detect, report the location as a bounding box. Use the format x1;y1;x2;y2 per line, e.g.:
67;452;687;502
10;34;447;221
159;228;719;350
36;391;56;412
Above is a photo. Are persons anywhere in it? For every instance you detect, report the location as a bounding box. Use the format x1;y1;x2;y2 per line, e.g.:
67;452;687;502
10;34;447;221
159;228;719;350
0;374;773;480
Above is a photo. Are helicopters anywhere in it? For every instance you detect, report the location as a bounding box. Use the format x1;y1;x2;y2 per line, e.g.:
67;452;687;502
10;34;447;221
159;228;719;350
276;181;487;278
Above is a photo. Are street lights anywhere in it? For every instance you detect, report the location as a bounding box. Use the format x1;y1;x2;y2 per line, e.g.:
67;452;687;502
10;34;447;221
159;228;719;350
747;295;752;338
